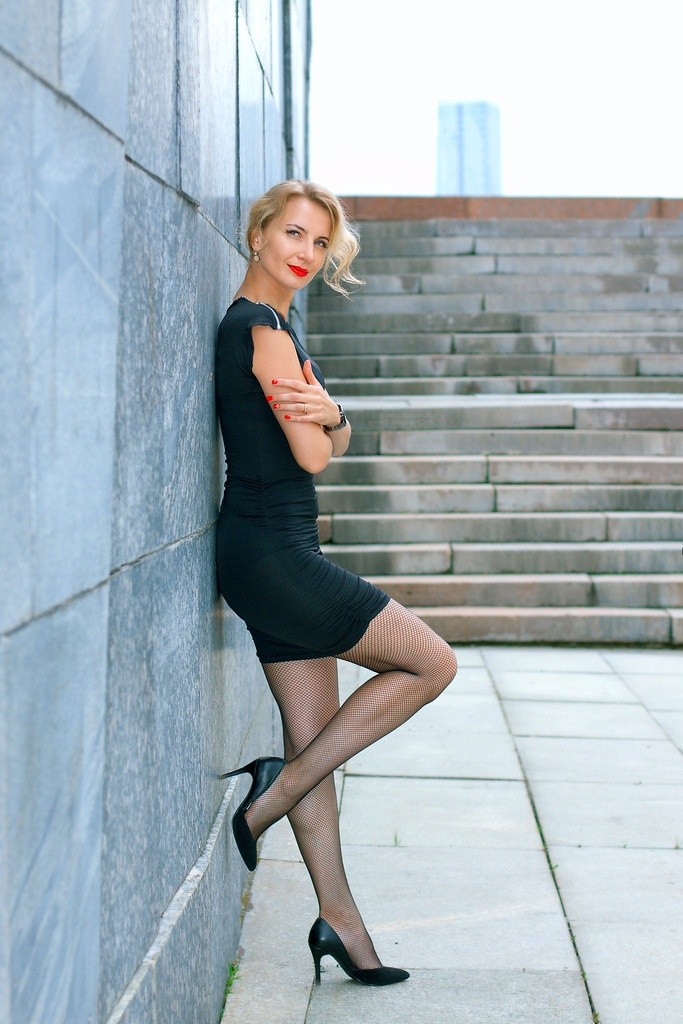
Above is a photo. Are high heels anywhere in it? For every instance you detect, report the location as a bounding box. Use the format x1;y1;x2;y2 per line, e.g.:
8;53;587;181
309;919;409;986
218;754;285;875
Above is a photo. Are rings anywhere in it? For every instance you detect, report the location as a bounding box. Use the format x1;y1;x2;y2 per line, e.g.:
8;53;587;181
302;404;306;413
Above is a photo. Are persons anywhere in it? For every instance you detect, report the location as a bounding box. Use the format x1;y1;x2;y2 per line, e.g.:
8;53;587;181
213;182;459;984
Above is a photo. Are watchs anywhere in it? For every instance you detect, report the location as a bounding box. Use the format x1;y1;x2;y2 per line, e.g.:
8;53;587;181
321;403;346;432
